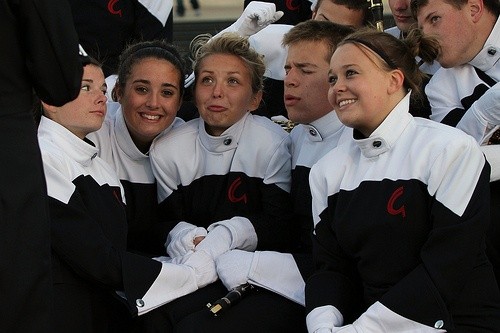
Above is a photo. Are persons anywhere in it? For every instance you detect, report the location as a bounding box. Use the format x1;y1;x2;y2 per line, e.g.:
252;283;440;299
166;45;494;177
0;0;83;333
71;0;500;286
149;37;305;333
33;44;129;333
305;27;500;333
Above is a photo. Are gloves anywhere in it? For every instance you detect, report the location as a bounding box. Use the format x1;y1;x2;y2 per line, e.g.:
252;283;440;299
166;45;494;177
216;250;253;290
475;81;500;126
207;1;283;54
307;306;342;331
